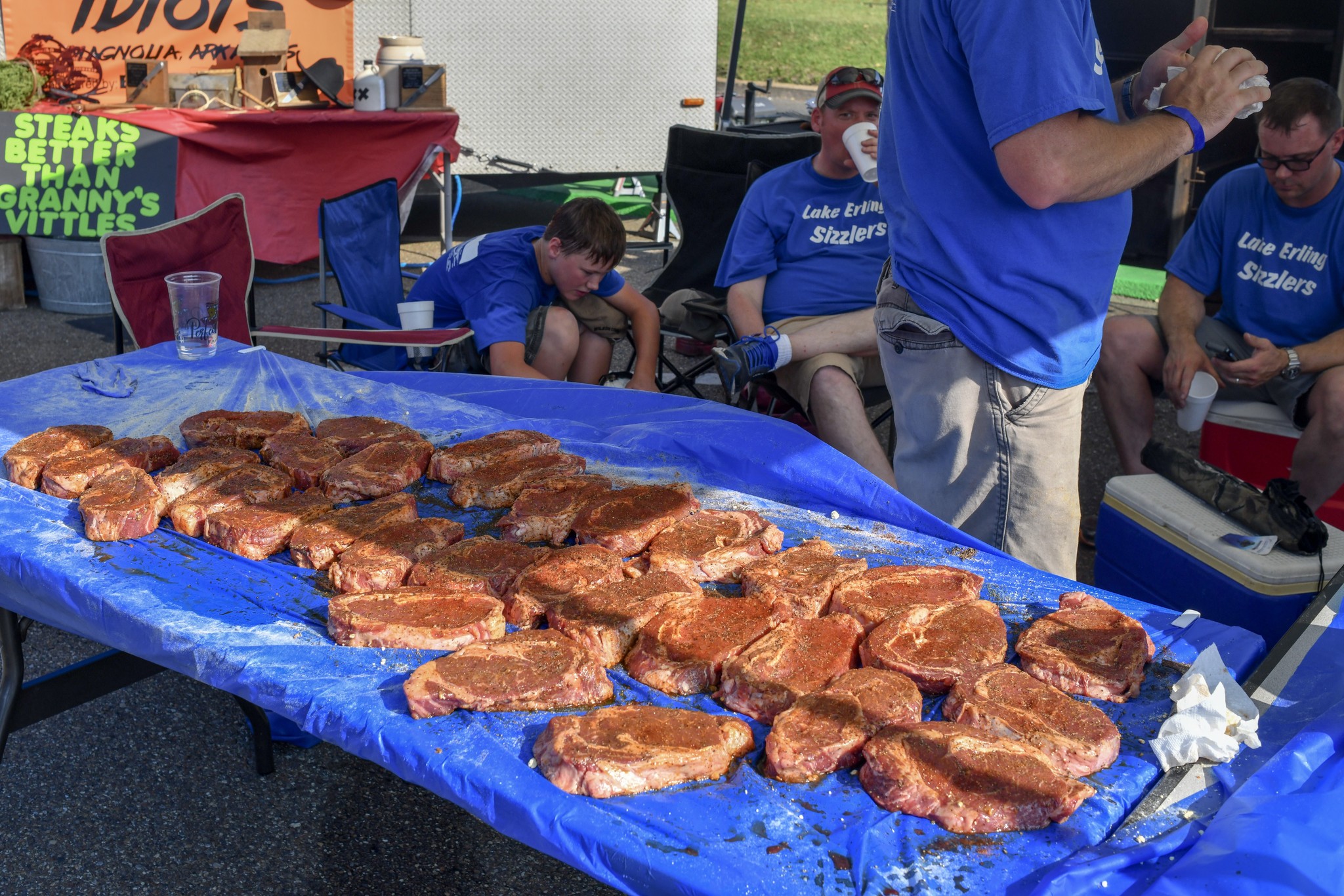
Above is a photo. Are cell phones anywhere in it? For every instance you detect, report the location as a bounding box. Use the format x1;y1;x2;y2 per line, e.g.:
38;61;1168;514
1204;341;1242;363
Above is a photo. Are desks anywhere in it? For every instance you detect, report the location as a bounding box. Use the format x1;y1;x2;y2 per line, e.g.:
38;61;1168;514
1;96;464;258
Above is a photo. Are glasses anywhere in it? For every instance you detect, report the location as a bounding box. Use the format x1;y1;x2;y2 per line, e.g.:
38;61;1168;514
817;66;884;108
1254;130;1335;171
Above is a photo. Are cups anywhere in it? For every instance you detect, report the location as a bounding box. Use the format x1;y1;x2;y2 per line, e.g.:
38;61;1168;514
1176;371;1219;432
841;121;880;183
163;271;221;361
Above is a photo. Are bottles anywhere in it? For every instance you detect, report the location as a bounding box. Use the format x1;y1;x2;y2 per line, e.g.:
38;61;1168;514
352;58;385;111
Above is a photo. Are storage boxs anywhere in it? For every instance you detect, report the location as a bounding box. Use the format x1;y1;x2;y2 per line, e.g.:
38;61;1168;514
1094;466;1344;652
1201;398;1342;532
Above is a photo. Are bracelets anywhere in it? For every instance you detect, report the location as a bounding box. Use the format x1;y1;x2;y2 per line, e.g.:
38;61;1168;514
1120;71;1143;119
1151;105;1206;153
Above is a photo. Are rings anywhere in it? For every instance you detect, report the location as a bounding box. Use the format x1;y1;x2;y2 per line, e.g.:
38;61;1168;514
1236;378;1240;384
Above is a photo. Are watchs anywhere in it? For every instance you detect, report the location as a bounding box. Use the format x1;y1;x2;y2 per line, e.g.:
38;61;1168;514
1281;346;1301;381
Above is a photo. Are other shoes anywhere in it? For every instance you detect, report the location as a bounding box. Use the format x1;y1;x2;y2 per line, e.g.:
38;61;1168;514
1078;514;1098;548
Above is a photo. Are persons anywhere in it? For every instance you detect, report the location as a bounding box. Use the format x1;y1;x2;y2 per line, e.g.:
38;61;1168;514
1078;77;1344;550
384;193;664;394
712;67;898;490
874;0;1273;582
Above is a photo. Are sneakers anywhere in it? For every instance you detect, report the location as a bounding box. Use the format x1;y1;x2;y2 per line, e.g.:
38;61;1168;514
710;333;778;397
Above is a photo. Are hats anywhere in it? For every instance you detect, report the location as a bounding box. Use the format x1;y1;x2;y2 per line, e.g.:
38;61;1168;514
816;66;884;110
296;50;355;109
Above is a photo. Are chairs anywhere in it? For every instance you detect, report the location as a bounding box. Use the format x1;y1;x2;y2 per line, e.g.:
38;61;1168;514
620;122;822;418
100;190;257;356
0;330;1344;896
741;364;897;473
314;176;471;374
